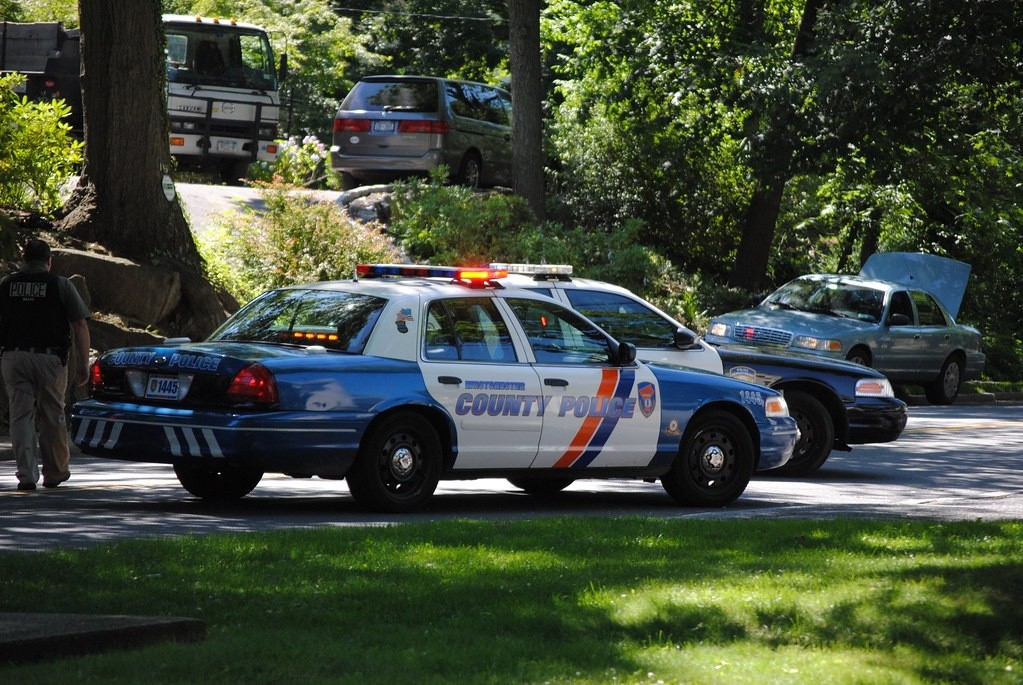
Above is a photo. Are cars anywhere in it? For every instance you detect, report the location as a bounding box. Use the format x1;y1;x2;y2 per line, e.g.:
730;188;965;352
71;263;800;518
703;250;986;406
488;258;908;479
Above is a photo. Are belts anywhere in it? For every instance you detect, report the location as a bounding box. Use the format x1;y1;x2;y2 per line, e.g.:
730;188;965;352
3;344;57;355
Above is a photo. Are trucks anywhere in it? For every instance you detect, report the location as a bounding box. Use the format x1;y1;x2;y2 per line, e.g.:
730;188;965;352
1;12;290;180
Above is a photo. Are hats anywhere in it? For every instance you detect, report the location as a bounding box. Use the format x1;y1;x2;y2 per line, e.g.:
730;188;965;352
23;240;50;260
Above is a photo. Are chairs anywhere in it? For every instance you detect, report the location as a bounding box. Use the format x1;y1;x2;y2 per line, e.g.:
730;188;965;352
890;295;911;317
192;40;225;75
474;305;527;343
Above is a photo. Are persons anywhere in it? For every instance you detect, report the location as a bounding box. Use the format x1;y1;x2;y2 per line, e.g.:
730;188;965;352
0;239;90;491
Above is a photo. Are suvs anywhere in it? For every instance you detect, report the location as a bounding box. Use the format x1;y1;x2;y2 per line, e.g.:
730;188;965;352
328;73;564;195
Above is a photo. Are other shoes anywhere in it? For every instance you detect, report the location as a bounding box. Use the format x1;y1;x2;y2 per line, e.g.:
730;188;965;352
18;482;36;490
42;470;70;487
